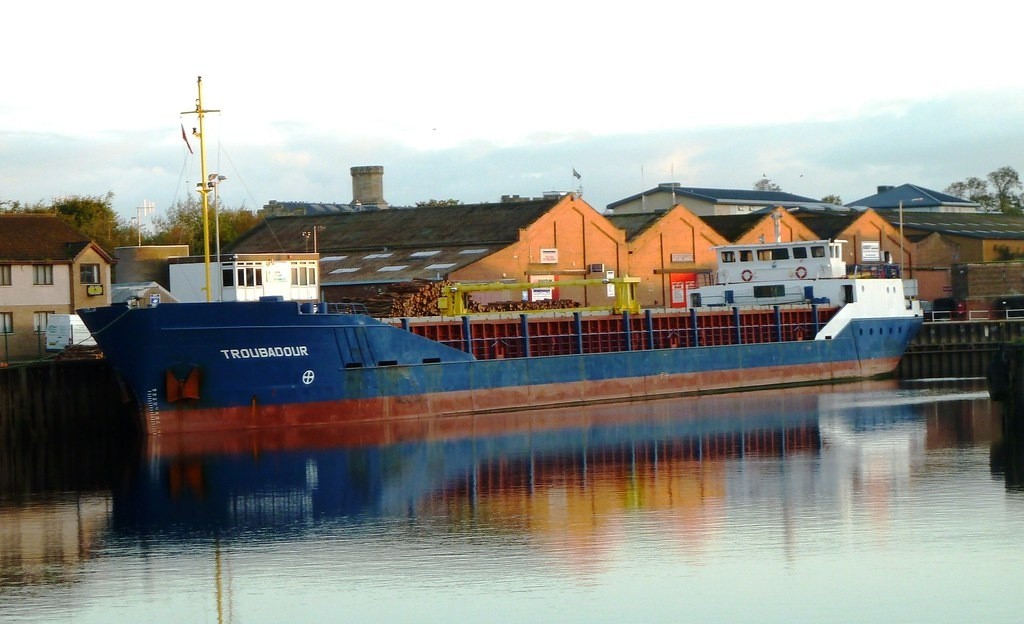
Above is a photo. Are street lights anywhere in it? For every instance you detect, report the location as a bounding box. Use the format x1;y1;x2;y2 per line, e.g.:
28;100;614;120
197;172;228;302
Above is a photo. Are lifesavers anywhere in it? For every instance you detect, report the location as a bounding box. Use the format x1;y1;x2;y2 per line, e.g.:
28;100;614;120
795;266;807;278
741;270;752;281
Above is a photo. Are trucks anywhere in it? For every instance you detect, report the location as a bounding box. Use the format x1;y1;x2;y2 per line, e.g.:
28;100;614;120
45;313;97;351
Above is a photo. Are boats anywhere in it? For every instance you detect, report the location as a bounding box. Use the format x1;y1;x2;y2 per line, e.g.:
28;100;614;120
71;75;922;436
